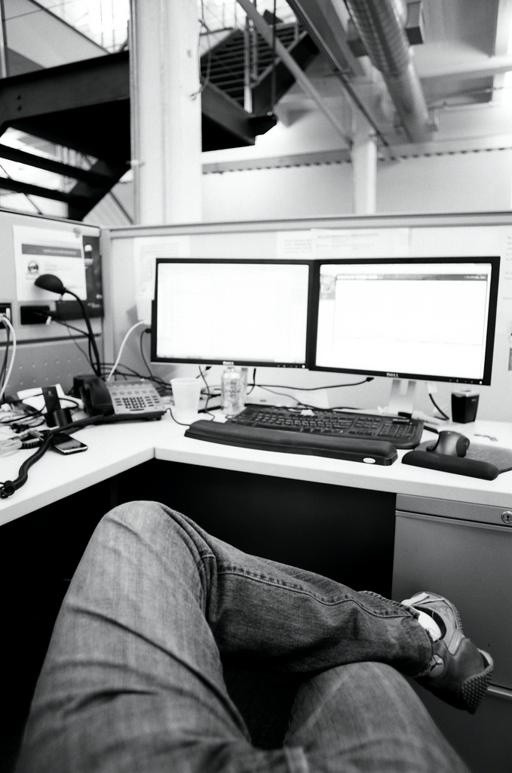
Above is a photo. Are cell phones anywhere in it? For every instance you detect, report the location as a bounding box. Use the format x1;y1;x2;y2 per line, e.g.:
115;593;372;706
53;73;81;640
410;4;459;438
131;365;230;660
50;434;90;452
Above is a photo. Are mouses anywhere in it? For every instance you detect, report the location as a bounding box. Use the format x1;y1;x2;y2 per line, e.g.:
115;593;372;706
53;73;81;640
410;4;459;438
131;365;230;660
432;430;471;458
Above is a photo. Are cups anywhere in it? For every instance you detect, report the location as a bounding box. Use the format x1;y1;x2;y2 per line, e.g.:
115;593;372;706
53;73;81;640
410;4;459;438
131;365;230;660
169;375;202;419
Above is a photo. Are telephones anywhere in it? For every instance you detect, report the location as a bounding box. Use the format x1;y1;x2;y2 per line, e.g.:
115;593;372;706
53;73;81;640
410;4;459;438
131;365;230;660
74;375;167;424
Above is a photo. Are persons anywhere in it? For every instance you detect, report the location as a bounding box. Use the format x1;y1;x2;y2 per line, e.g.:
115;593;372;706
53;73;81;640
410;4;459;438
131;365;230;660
17;499;494;773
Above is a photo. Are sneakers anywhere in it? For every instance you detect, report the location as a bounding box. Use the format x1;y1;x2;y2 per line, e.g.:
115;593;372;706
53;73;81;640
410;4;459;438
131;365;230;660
400;589;493;716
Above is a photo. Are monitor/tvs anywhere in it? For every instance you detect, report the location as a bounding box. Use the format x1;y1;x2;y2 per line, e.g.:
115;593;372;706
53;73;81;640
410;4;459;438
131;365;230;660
151;258;310;370
313;255;501;413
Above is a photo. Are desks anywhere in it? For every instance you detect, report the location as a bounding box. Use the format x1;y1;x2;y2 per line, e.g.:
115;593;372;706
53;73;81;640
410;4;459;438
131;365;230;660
0;399;512;768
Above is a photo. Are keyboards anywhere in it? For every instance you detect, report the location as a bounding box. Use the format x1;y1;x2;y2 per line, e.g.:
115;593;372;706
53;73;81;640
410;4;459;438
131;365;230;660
227;402;425;449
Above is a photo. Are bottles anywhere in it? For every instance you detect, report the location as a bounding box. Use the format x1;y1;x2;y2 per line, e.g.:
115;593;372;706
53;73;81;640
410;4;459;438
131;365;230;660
221;367;246;415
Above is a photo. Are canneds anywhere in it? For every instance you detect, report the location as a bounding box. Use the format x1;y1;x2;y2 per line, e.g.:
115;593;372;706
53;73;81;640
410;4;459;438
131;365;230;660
222;367;247;416
